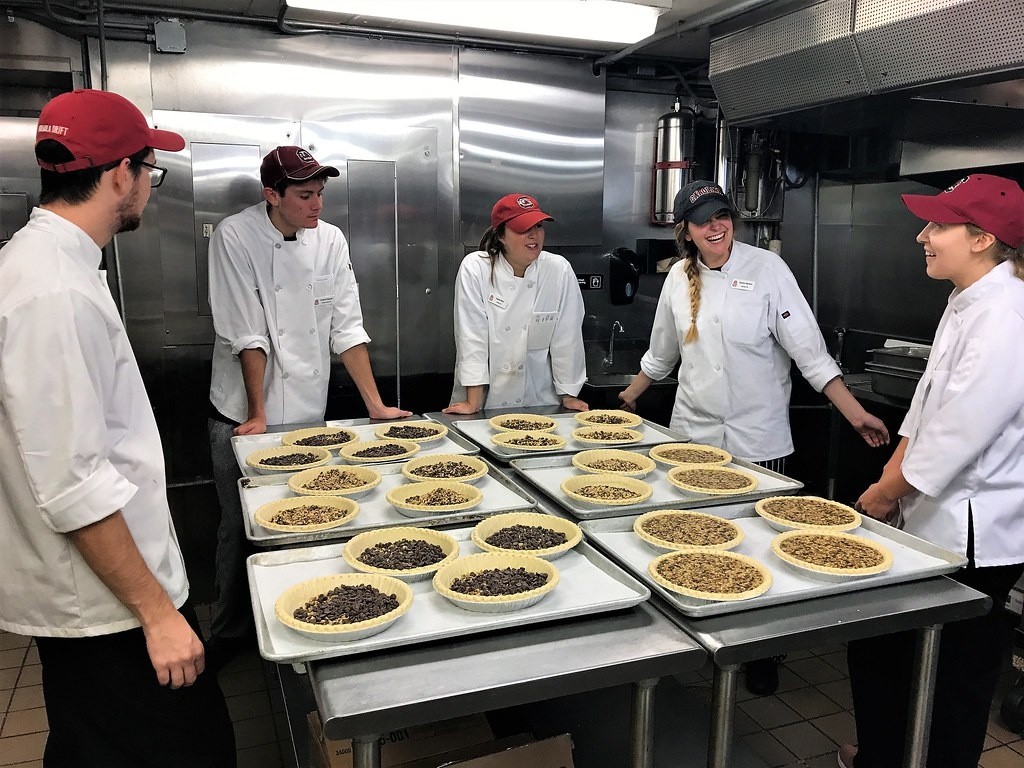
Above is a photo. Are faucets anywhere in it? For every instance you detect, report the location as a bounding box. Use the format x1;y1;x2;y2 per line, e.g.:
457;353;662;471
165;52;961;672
602;320;625;369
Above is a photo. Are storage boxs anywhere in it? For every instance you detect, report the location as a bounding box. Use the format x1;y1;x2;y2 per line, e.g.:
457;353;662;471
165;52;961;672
306;710;495;768
394;732;575;768
864;368;920;401
872;348;928;370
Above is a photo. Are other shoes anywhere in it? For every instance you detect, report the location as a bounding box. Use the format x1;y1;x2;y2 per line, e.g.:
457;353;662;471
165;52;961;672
838;742;859;768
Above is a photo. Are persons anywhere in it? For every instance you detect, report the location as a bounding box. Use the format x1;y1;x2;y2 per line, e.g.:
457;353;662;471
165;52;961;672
619;182;890;477
837;172;1024;768
443;195;590;414
0;89;237;768
205;146;413;671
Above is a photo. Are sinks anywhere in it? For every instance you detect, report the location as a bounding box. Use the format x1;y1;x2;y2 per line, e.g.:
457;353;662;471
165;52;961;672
584;372;680;387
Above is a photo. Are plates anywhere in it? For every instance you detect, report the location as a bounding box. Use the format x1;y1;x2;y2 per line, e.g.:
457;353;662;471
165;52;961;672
288;465;382;499
572;449;656;479
246;446;332;474
386;482;483;518
572;427;644;447
281;426;358;455
560;474;653;509
649;443;732;466
274;495;892;642
338;440;421;464
574;410;643;429
254;496;359;535
490;413;558;433
402;454;489;484
666;466;759;498
375;421;448;443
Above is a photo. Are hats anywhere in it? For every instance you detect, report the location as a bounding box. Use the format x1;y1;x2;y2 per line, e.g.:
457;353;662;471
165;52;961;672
673;180;737;225
491;193;556;233
36;88;185;172
260;146;340;190
900;174;1024;249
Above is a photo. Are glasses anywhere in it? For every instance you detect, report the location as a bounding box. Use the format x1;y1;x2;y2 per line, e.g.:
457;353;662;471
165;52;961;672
105;159;169;188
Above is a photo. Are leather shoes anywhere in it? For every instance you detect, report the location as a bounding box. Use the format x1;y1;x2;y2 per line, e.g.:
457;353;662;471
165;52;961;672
745;657;779;693
204;632;248;672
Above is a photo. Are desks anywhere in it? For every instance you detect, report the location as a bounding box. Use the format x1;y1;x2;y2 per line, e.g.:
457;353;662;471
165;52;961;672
423;404;994;768
265;414;709;768
